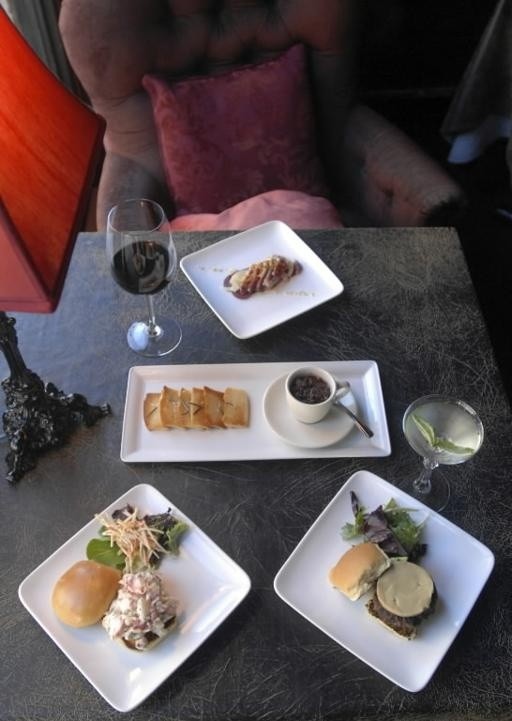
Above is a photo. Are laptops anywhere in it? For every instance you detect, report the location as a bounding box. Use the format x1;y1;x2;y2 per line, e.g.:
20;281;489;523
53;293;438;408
51;560;120;628
329;544;390;603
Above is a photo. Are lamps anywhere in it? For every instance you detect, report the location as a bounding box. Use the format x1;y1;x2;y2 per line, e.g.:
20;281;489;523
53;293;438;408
59;0;472;235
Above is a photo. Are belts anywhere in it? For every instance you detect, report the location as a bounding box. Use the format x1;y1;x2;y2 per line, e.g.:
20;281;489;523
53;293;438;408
394;395;483;514
106;199;186;357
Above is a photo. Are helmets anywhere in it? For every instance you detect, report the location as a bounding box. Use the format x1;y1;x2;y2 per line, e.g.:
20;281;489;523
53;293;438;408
15;482;252;715
263;375;357;453
120;355;391;464
269;469;494;693
181;221;351;347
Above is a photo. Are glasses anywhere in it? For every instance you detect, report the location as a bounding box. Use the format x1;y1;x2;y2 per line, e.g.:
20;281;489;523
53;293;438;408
0;8;108;484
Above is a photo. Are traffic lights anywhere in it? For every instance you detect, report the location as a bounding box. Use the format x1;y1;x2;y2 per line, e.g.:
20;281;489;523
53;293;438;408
141;43;333;216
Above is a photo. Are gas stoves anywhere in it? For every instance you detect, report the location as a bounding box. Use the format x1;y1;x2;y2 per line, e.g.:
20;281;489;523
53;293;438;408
101;571;184;653
364;561;438;642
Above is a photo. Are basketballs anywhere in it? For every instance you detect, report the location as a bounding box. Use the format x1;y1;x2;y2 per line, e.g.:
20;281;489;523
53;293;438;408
142;385;250;431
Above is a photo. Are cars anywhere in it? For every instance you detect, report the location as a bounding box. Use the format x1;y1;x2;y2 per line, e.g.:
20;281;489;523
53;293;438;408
284;364;351;425
